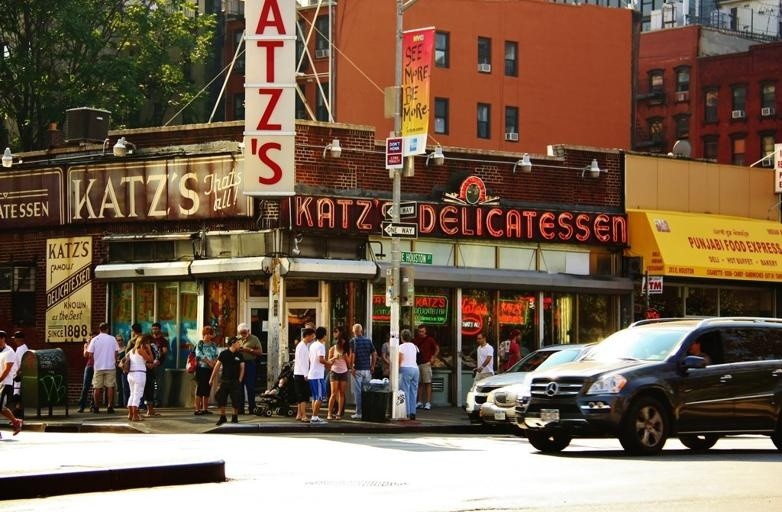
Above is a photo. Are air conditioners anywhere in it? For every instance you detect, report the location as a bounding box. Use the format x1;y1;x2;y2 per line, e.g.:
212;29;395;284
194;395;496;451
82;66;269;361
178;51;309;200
761;107;775;118
504;132;519;143
315;48;330;59
477;63;491;73
731;110;745;119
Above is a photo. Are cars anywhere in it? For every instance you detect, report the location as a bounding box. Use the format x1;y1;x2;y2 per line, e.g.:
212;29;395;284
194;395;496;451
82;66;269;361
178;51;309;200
466;338;598;430
428;343;530;369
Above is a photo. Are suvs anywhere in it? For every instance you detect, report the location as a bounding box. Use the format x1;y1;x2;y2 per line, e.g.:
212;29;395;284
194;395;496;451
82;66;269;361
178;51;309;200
524;314;781;454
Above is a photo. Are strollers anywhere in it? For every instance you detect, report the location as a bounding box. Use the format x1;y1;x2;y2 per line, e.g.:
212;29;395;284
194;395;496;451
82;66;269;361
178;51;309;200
254;361;295;417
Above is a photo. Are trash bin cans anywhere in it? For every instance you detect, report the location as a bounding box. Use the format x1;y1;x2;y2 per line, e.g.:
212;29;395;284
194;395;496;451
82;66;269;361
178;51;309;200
361;381;393;423
20;348;68;419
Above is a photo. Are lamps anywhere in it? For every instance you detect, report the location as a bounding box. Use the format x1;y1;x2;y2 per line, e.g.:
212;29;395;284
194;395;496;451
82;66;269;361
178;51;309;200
425;145;445;166
512;153;532;173
114;136;137;156
2;147;23;168
581;158;600;180
322;139;341;161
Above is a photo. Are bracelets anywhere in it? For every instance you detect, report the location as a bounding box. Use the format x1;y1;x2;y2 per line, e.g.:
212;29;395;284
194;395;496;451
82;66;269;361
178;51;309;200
480;365;483;368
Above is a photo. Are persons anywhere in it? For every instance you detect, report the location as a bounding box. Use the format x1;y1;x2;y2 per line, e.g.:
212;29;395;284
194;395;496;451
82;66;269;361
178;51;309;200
504;329;522;372
79;322;168;421
196;322;262;425
470;331;495;393
11;330;29;419
0;330;24;439
293;324;438;425
688;339;711;366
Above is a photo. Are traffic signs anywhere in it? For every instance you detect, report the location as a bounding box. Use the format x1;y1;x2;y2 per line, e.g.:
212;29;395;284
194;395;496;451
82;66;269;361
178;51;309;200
380;221;418;240
401;252;432;265
385;138;403;153
384;153;403;168
384;201;418;220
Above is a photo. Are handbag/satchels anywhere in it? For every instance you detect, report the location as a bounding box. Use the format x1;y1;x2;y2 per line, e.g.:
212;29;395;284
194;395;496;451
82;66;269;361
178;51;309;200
186;352;198;373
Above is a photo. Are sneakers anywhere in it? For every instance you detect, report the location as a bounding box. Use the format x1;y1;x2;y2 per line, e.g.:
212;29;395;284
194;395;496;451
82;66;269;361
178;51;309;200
13;419;23;435
416;401;424;408
424;402;431;410
216;415;226;425
296;409;362;424
232;414;238;423
